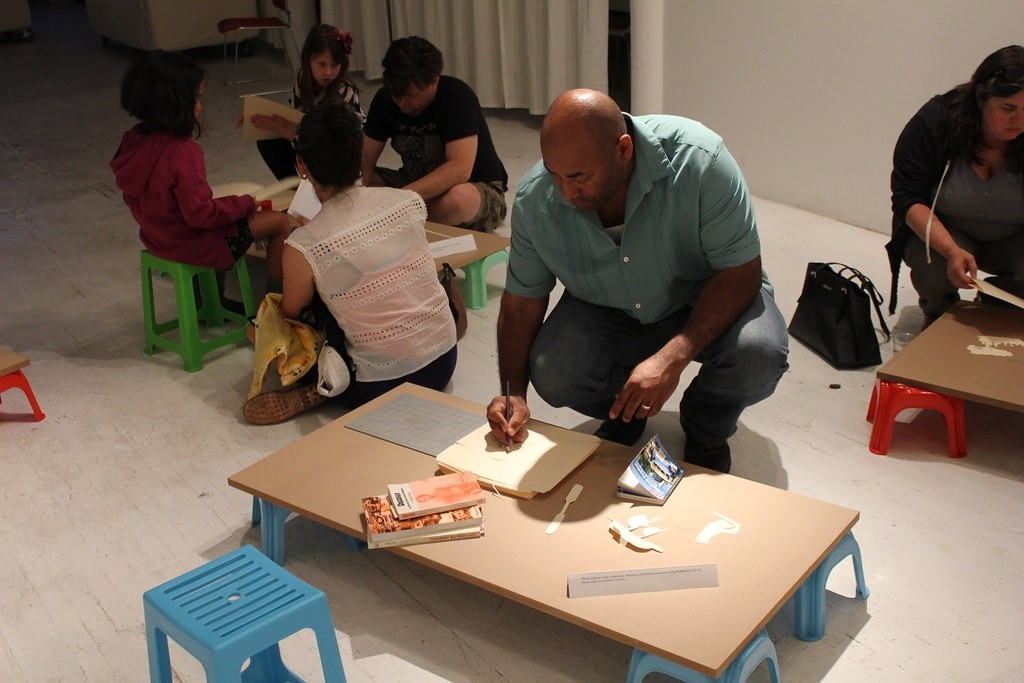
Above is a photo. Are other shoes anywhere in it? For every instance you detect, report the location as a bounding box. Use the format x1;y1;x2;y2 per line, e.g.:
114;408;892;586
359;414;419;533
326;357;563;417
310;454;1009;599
919;296;939;332
243;384;329;426
594;412;648;446
682;430;731;473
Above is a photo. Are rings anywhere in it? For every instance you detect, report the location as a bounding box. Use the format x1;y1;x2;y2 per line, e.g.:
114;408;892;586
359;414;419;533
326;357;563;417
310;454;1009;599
641;404;651;410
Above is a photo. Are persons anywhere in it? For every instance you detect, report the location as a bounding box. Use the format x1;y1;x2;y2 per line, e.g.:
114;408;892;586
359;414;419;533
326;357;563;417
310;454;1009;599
414;482;482;504
236;24;368;197
885;45;1024;333
110;50;292;330
360;36;509;232
486;89;789;474
243;99;458;426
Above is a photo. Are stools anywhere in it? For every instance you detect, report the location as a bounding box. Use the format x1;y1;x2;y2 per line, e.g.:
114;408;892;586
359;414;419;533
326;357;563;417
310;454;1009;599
626;627;782;683
140;249;255;372
866;377;967;459
143;544;346;683
0;369;46;422
251;496;364;566
795;530;870;642
459;248;510;309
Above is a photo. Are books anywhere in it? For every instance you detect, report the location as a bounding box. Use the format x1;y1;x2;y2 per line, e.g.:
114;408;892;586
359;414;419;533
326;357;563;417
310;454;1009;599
617;434;684;505
362;472;486;550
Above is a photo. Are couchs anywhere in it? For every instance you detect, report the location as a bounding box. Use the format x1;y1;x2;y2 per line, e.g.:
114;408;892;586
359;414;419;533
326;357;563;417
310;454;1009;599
86;0;260;61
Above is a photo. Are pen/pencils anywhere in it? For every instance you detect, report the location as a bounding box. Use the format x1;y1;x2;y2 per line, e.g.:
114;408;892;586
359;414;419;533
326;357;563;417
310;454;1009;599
506;381;509;454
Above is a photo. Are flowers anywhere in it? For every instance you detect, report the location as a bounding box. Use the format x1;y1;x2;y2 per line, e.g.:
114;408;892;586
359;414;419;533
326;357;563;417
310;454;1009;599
333;28;354;53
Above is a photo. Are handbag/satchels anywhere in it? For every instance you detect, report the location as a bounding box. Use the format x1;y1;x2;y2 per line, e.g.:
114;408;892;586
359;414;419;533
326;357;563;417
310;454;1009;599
787;261;892;369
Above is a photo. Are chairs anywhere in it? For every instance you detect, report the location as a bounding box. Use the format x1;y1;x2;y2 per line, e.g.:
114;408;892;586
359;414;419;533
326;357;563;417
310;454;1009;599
218;0;303;98
